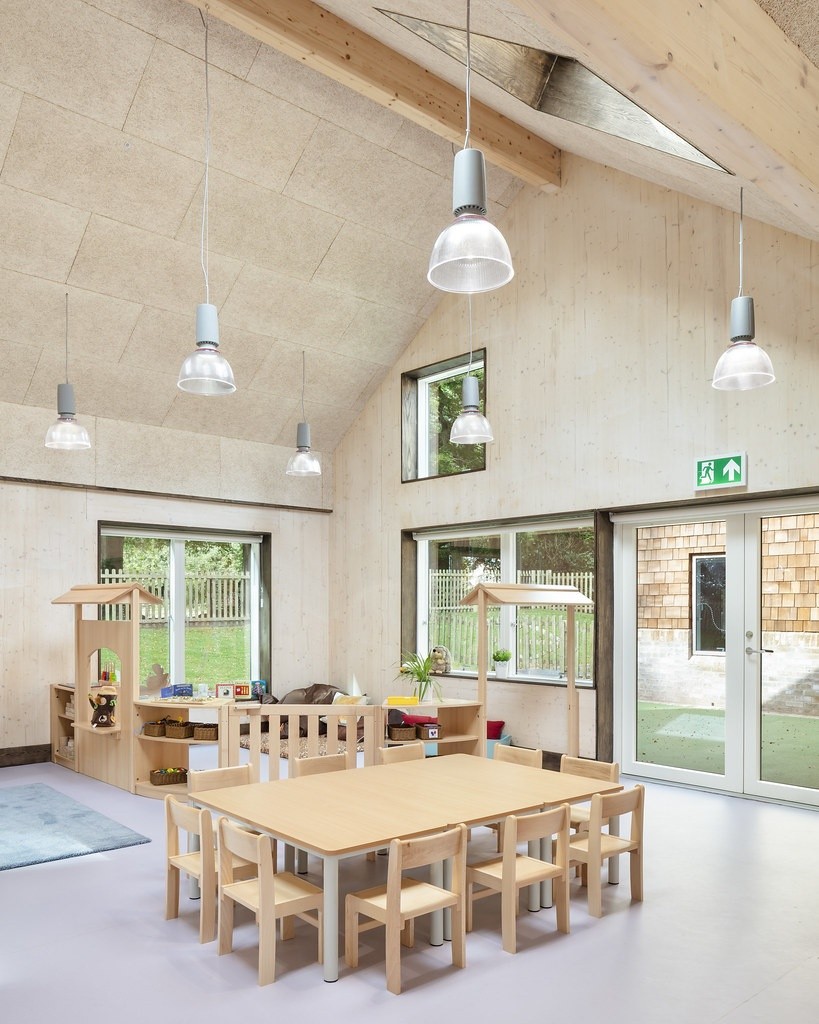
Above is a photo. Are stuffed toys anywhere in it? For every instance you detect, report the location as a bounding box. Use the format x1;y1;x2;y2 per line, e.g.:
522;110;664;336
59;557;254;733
429;645;451;673
89;694;116;726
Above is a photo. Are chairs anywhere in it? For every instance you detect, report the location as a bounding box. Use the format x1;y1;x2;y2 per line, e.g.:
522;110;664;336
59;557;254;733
160;741;646;997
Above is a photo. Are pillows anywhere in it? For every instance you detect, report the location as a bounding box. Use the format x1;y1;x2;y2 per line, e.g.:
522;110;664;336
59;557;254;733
319;692;371;726
487;720;505;739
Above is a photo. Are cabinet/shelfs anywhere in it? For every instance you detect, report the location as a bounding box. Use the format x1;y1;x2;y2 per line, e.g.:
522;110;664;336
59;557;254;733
381;698;485;758
49;680;120;772
133;696;234;803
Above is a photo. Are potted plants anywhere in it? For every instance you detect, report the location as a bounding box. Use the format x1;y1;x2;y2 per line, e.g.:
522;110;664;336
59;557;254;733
492;648;512;678
386;648;444;703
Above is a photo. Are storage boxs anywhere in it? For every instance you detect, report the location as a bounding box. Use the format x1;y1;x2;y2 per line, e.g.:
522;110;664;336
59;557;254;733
487;734;512;759
161;683;193;697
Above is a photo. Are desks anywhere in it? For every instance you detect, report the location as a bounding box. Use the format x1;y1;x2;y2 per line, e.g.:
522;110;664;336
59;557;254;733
186;751;625;983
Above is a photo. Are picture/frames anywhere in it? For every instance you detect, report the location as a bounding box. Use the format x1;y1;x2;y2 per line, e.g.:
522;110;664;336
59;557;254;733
215;683;235;700
234;685;251;699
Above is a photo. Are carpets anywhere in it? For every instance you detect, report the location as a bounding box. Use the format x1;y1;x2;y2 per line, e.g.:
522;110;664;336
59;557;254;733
0;780;151;870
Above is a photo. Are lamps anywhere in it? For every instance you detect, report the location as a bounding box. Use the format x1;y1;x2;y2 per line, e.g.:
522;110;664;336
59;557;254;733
43;0;777;475
